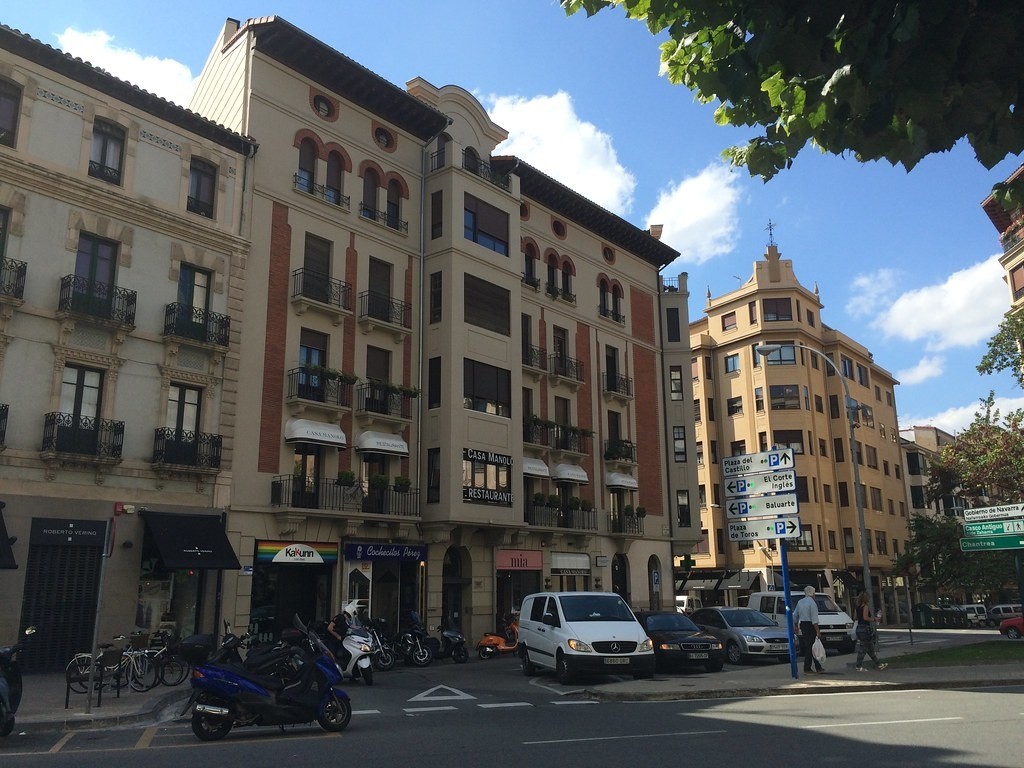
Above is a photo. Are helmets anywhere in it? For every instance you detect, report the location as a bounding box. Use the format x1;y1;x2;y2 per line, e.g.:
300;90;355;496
345;605;359;620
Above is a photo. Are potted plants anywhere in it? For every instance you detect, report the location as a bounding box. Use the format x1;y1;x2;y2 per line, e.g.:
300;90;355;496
370;474;411;493
301;363;359;385
334;471;356;487
371;379;422;399
622;504;647;518
533;493;593;513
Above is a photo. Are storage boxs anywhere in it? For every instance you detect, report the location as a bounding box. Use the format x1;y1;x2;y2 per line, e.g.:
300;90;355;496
280;628;302;646
179;633;215;664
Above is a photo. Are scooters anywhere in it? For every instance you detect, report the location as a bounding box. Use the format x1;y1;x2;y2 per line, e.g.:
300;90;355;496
180;615;353;741
476;610;525;660
307;614;376;685
203;616;305;678
355;608;470;672
0;624;39;737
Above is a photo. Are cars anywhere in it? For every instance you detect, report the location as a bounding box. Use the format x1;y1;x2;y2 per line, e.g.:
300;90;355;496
678;606;801;666
629;610;727;676
1000;616;1024;639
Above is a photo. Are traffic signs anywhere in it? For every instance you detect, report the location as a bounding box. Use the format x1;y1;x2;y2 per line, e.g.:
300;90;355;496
723;470;797;499
727;516;803;542
721;447;796;478
725;491;800;520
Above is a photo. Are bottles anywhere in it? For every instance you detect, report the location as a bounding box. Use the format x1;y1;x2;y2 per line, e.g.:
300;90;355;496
876;609;883;626
793;634;798;640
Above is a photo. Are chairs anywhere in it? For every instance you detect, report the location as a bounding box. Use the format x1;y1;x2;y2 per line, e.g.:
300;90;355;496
65;648;122;709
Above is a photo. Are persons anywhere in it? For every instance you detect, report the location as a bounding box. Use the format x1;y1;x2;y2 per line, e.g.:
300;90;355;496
855;591;889;671
327;605;369;683
793;585;825;676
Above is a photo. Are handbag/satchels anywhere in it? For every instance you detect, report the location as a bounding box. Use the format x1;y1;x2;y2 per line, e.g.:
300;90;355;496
850;620;858;641
812;635;827;665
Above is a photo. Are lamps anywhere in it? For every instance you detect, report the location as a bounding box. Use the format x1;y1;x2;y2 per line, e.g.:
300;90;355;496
595;577;601;588
122;540;134;549
544;577;551;589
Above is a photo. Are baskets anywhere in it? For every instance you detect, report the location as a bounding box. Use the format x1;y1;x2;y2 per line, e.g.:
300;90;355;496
131;633;151;650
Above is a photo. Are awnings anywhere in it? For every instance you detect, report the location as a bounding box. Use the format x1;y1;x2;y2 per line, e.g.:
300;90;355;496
773;571;830;588
675;572;759;591
140;511;241;573
285;417;347;451
522;457;549;479
552;463;589;486
355;430;409;459
832;571;865;589
606;472;638;492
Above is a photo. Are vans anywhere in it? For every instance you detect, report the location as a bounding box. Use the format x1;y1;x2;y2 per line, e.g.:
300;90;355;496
931;604;988;628
747;590;856;657
517;591;656;685
986;604;1024;628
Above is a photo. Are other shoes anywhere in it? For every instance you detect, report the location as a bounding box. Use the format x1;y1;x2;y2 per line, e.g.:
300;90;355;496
856;666;869;672
816;669;826;674
878;662;889;670
803;669;818;676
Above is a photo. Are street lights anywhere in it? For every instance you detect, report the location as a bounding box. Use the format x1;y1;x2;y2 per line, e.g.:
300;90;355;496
756;344;880;651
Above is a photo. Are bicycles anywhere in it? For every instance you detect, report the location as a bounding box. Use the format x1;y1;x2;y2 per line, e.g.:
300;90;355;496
65;629;192;694
